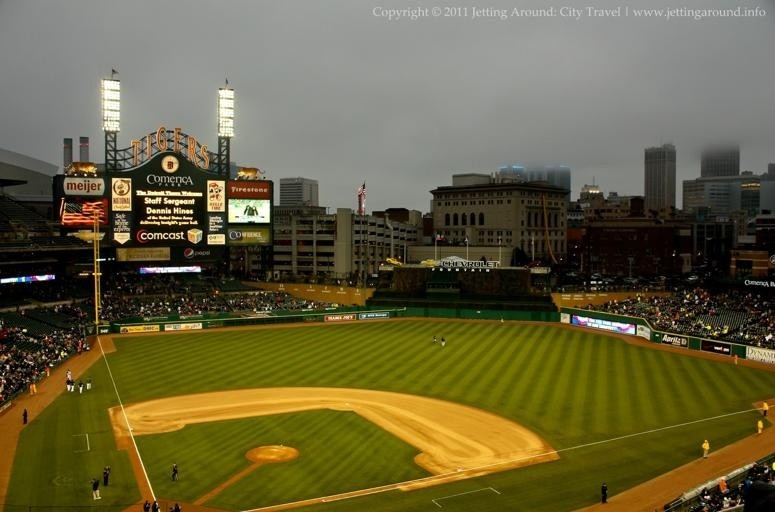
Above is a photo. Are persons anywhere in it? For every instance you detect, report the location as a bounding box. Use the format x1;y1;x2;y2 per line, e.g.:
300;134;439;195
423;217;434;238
0;238;774;512
